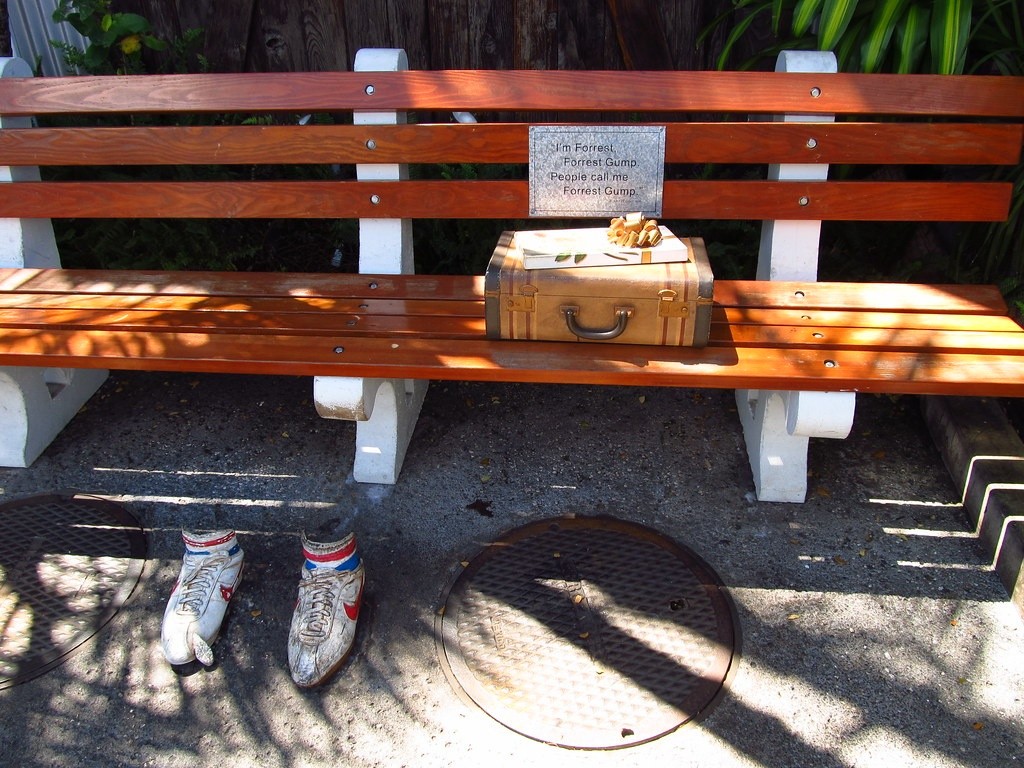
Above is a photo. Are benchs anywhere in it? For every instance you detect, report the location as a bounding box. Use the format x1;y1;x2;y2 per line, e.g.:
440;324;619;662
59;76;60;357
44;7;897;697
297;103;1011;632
0;42;1023;505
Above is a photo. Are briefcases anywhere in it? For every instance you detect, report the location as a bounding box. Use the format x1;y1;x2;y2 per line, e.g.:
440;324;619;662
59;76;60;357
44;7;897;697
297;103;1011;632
485;230;714;347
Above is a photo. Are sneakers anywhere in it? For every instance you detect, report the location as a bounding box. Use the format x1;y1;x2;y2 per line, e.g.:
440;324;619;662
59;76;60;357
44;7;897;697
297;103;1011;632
161;531;244;667
288;529;365;688
